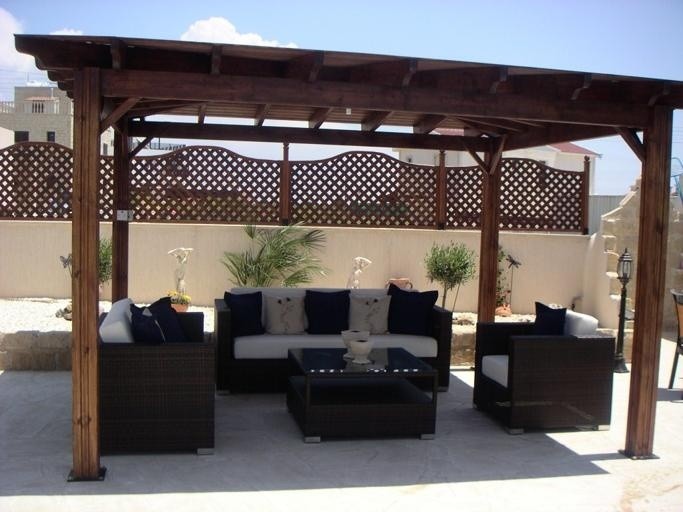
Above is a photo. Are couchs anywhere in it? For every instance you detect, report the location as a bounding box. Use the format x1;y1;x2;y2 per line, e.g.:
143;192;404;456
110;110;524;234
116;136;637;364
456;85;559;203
213;299;452;395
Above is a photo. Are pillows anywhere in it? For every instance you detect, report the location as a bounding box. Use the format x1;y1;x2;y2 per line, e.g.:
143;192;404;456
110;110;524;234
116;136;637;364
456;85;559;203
530;302;567;336
130;297;189;343
224;284;439;339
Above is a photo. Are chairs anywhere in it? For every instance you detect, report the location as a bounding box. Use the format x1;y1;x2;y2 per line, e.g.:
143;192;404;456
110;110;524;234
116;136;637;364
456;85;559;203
95;311;213;454
668;289;683;400
473;322;615;435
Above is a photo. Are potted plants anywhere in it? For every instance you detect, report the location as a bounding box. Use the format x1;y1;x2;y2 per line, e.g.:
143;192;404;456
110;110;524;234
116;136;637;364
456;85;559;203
167;291;191;313
